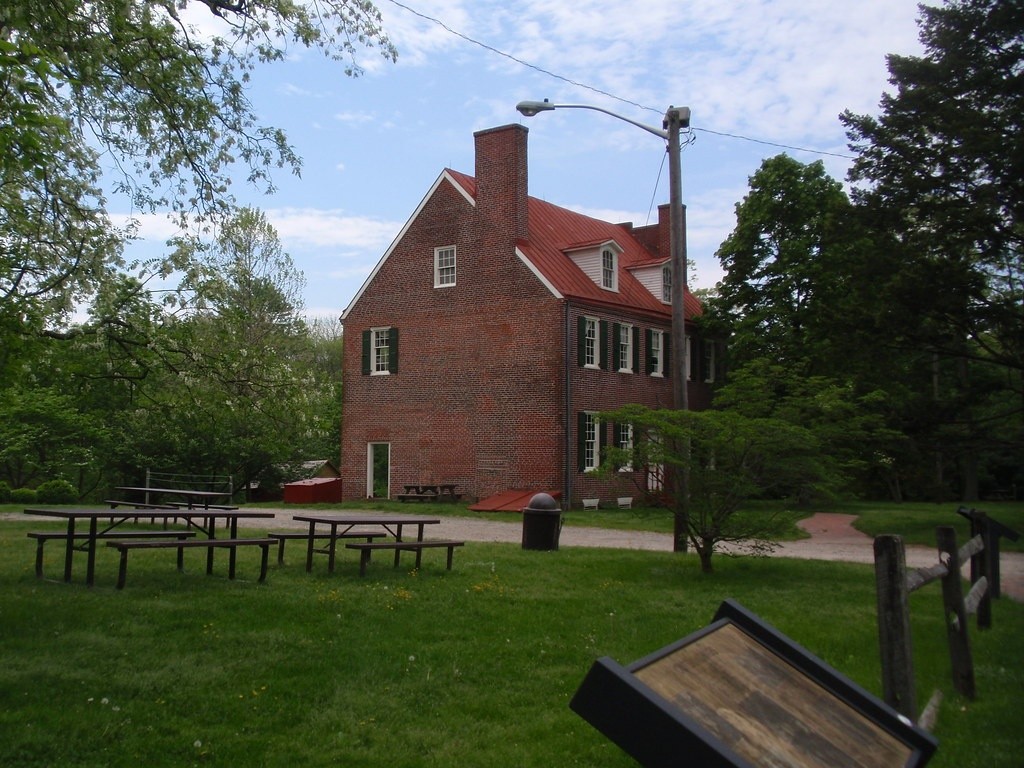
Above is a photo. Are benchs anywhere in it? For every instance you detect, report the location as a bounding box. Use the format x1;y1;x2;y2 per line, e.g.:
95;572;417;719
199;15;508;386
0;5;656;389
344;542;464;571
104;538;279;589
268;532;387;563
395;493;466;502
107;500;240;529
26;531;197;583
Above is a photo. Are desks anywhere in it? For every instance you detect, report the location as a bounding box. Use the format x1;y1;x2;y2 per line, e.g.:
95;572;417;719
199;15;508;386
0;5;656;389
24;507;276;587
293;515;441;573
117;486;233;528
404;485;459;502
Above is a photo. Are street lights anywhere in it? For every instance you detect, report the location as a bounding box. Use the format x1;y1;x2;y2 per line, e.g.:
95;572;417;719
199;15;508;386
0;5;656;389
516;98;690;551
